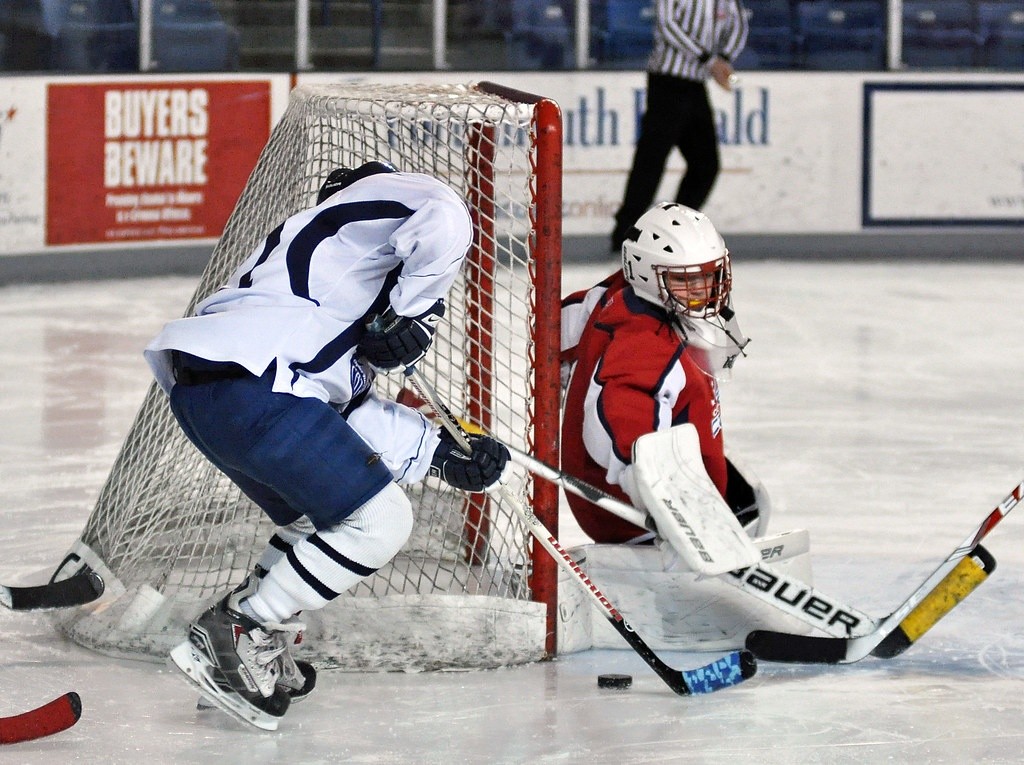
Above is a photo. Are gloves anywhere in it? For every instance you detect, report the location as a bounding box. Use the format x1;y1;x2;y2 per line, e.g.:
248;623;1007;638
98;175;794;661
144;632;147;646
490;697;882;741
424;425;513;494
357;302;445;376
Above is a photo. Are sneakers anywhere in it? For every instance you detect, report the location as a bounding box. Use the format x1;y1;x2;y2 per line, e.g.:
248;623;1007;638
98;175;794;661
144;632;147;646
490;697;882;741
168;568;316;732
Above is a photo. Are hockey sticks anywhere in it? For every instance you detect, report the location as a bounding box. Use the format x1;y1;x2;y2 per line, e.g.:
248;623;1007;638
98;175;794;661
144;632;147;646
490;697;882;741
745;479;1023;665
366;315;757;699
397;388;998;662
0;572;106;613
0;690;82;744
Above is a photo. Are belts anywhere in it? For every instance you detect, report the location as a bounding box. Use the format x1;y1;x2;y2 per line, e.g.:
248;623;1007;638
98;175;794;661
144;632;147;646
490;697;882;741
174;371;249;387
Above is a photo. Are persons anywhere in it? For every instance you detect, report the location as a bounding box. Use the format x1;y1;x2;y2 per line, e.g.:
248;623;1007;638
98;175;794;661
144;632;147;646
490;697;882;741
558;202;770;546
608;0;749;260
145;162;511;733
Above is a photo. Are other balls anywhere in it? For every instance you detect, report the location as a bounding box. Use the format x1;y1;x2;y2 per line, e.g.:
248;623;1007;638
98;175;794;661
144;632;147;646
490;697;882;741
597;672;635;692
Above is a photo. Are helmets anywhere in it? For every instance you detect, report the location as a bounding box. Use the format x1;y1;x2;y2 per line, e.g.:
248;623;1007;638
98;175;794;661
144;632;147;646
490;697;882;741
622;201;732;350
316;160;395;207
